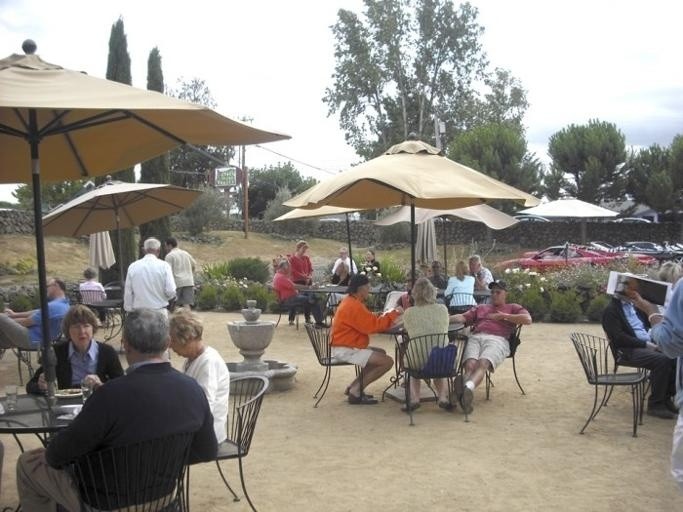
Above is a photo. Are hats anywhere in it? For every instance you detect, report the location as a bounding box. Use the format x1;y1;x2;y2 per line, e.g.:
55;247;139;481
488;280;508;291
346;275;368;292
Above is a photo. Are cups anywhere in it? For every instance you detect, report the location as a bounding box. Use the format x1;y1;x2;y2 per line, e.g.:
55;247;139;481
79;379;93;405
4;386;17;412
3;302;9;313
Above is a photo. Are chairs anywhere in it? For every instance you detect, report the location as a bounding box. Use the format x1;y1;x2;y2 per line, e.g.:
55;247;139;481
399;332;470;426
570;332;648;437
605;341;652;407
469;319;525;399
305;321;363;409
11;279;125;386
63;430;194;512
270;277;501;330
186;373;270;512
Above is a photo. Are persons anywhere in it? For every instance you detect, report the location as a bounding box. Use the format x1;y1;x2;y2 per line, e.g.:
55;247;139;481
286;241;314;326
446;279;532;413
162;238;198;312
657;259;683;287
468;255;493;304
77;268;108;325
395;276;458;411
393;269;427;342
2;276;71;351
601;290;679;420
17;308;219;512
331;247;359;287
24;305;125;388
319;274;405;404
361;248;381;279
271;260;331;330
119;235;179;318
162;309;231;447
619;271;682;487
442;261;478;313
427;261;449;293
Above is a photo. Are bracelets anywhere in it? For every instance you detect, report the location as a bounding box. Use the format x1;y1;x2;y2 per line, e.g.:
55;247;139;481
647;310;666;323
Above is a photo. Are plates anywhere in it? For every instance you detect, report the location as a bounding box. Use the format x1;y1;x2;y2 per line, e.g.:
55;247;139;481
326;284;338;288
54;389;84;400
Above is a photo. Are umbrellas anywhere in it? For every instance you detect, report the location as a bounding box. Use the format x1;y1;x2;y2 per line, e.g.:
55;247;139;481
515;196;620;266
86;231;118;296
377;207;520;271
273;195;401;272
281;131;529;299
1;34;296;397
37;172;206;298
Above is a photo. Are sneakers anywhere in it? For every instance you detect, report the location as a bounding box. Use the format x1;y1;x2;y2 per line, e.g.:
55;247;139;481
647;400;676;418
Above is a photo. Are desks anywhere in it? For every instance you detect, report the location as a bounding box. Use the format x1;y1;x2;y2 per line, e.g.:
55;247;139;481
382;323;465;406
0;390;96;512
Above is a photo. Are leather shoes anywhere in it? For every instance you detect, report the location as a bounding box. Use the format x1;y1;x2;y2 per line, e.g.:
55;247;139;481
345;376;474;415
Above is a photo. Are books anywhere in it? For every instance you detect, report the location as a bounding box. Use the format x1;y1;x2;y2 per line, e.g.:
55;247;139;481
605;269;674;307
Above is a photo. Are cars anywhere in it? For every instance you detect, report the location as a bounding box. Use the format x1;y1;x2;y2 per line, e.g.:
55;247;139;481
611;217;650;223
492;238;683;276
514;215;549;222
